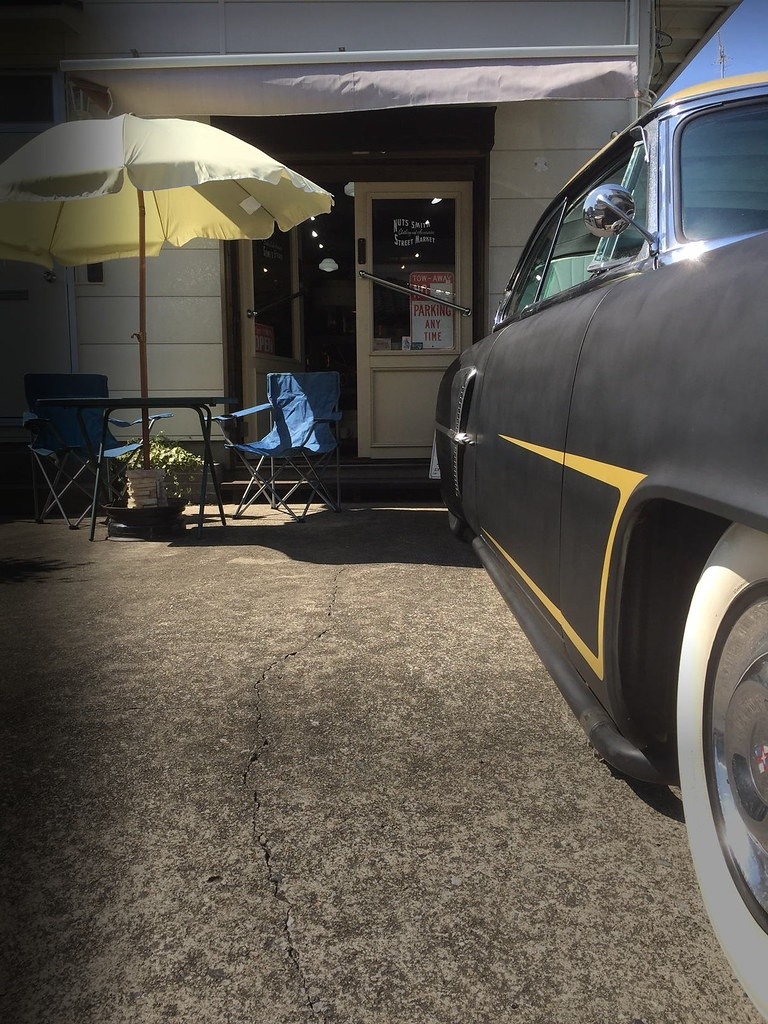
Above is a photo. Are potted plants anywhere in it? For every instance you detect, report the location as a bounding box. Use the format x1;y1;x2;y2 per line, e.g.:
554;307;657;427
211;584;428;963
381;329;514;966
116;437;225;508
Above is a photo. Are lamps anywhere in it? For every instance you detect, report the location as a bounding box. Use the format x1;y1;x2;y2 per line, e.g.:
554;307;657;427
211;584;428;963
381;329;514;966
319;256;339;272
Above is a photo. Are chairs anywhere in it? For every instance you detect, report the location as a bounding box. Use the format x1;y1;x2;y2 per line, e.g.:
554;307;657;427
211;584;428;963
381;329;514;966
212;370;341;525
20;372;176;532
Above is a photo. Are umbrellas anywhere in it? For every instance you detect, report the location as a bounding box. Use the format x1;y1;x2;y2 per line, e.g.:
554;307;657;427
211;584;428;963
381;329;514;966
0;113;335;471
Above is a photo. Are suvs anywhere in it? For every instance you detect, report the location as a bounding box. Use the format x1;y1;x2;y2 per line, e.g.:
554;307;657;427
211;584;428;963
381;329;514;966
431;64;768;1024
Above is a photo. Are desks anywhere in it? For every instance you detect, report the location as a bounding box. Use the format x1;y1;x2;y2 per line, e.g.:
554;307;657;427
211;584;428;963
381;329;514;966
34;395;241;542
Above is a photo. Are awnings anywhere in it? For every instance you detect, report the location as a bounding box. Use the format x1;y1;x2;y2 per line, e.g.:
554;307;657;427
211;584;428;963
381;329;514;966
60;47;650;118
